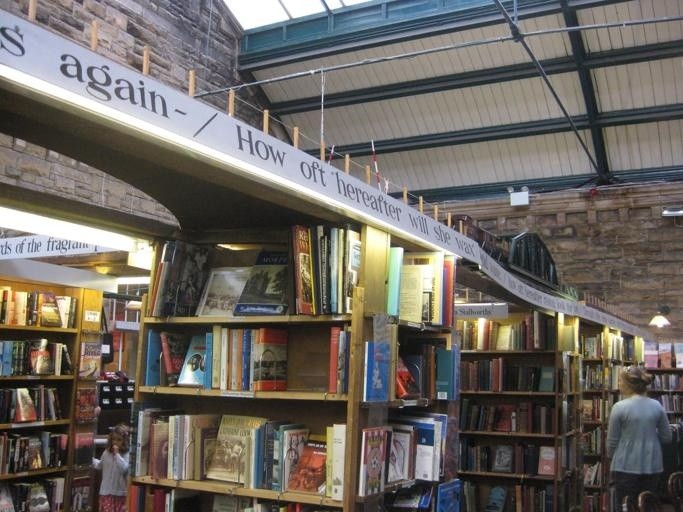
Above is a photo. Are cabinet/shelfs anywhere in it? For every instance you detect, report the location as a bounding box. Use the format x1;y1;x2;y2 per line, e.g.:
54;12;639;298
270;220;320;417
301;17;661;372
0;226;683;512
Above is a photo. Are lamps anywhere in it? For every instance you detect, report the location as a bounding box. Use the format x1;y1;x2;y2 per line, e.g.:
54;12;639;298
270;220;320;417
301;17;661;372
648;307;671;329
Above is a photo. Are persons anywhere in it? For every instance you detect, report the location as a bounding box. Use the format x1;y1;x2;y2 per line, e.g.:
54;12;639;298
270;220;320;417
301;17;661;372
90;424;129;512
604;363;674;511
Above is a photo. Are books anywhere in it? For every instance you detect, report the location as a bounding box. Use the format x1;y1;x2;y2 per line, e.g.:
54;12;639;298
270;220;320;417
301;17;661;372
356;225;463;510
2;285;76;510
579;327;647;511
643;339;682;425
69;288;103;511
455;310;573;512
144;221;361;395
127;402;346;511
